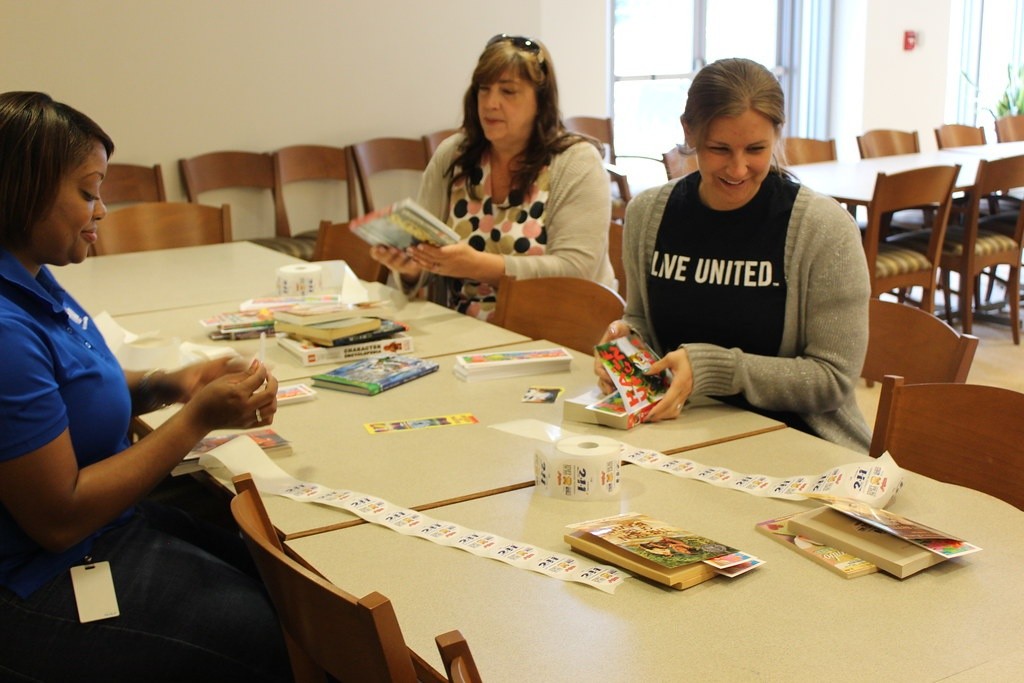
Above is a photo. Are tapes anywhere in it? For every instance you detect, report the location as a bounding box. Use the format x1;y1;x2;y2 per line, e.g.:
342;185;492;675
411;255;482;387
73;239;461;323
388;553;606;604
535;434;621;499
277;259;368;304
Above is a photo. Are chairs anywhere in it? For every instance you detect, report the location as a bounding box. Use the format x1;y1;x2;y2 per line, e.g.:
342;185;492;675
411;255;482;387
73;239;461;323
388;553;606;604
46;115;1024;683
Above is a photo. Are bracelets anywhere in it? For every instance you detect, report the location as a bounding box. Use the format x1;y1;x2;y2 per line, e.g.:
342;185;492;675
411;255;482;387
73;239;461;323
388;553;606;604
138;369;176;411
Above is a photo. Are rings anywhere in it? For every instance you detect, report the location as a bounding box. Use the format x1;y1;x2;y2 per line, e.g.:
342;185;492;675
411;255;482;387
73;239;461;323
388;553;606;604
431;262;436;268
256;408;263;422
677;404;682;410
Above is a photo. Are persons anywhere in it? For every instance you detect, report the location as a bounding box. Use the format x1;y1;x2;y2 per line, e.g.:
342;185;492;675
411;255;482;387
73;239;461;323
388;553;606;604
369;33;621;332
593;57;874;459
1;90;287;682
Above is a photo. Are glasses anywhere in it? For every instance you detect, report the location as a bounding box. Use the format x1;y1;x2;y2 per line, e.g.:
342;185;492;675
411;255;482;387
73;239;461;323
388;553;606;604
487;34;547;76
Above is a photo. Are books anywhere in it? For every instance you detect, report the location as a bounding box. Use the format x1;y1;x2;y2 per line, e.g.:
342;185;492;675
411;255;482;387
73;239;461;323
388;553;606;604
169;428;292;482
562;511;747;591
309;349;439;397
206;304;416;368
562;335;675;430
754;502;970;579
348;195;460;252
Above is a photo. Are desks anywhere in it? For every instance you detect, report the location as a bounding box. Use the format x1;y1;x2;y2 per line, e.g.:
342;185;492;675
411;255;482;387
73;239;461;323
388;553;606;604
89;295;535;411
944;140;1023;194
284;381;1024;681
46;241;367;329
141;338;787;534
785;150;999;241
